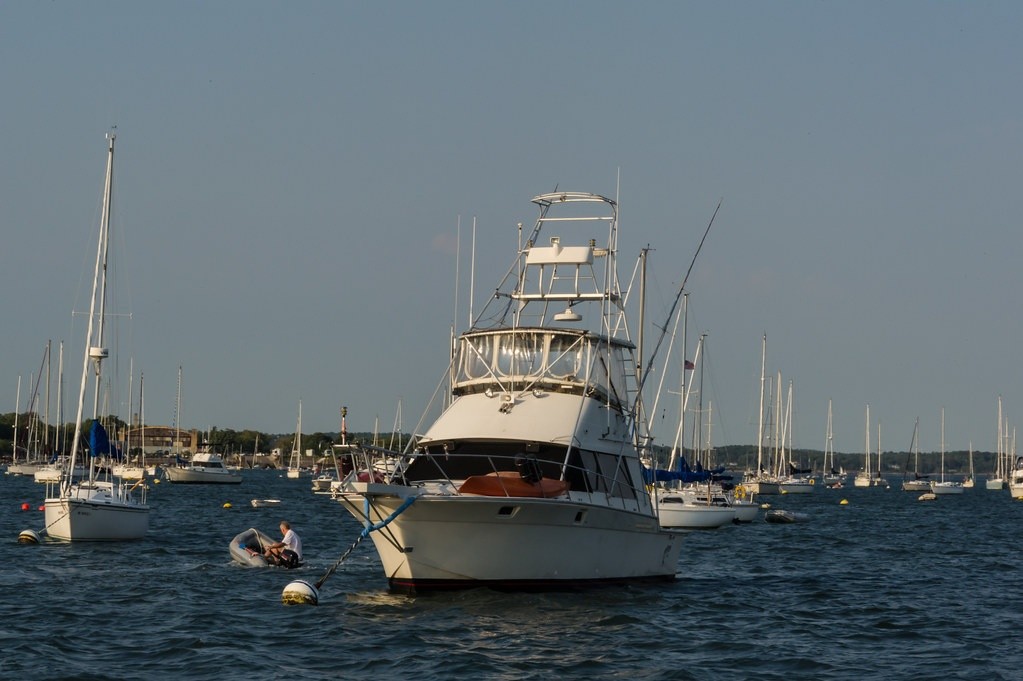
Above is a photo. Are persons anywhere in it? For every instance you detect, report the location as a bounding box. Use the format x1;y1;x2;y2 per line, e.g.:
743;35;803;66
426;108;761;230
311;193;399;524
263;520;304;564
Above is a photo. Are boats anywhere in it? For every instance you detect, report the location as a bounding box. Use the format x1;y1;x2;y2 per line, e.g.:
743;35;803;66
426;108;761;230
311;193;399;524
332;192;682;582
230;529;307;571
251;498;283;508
166;450;243;485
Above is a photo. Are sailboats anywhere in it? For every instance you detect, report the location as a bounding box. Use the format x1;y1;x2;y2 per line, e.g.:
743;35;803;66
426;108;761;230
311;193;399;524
6;339;163;483
45;124;154;543
645;325;1023;530
225;398;407;489
